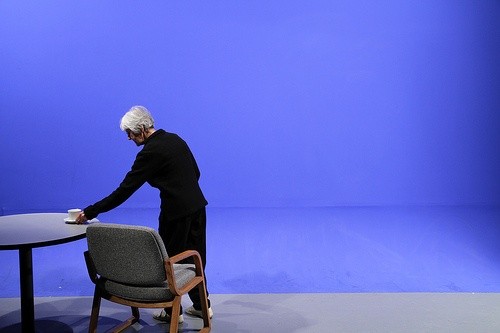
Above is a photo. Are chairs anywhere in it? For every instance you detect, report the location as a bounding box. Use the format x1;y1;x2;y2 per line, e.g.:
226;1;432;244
84;224;211;333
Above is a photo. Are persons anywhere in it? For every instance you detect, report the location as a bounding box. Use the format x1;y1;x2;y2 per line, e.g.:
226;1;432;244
75;106;214;323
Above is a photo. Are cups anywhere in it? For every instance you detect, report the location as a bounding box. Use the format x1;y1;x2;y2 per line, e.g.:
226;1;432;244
68;209;81;220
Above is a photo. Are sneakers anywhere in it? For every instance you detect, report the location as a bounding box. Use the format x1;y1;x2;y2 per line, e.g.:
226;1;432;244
153;308;184;324
185;305;213;319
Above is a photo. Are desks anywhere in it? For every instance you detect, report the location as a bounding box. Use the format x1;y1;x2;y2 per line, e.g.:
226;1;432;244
0;213;100;333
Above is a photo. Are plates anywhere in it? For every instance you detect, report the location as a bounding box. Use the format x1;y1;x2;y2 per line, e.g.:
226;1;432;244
64;217;77;222
